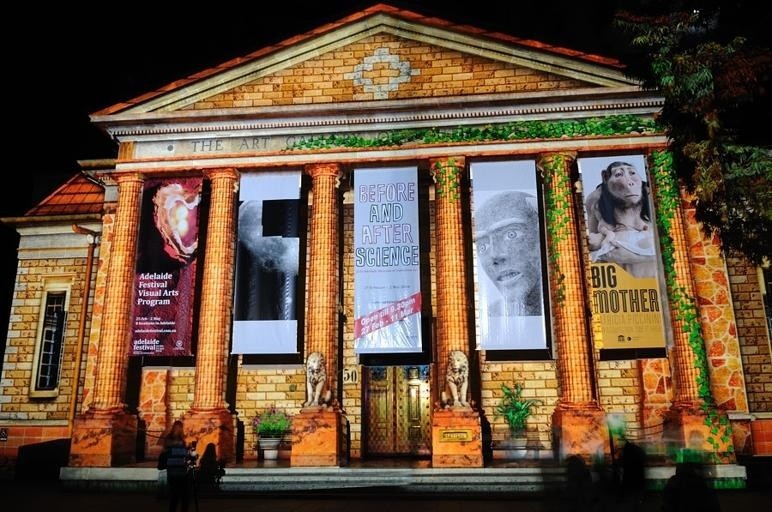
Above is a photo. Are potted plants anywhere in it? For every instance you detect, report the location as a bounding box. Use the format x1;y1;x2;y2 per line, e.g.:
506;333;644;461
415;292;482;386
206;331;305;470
493;384;545;450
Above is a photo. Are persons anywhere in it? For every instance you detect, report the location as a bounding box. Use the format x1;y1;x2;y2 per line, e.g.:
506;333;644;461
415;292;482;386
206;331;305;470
472;192;542;316
157;420;226;487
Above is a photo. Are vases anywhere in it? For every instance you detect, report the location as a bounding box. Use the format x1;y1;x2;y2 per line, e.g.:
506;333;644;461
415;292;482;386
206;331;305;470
257;438;281;452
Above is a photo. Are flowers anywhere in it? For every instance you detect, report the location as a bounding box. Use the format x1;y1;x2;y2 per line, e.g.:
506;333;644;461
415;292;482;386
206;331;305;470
250;404;292;437
